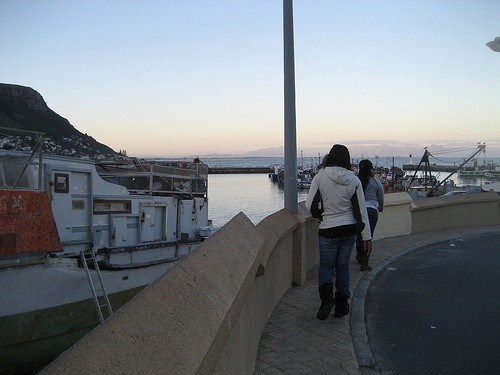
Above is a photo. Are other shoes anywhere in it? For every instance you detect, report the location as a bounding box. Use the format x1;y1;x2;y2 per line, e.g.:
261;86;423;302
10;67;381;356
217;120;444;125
361;266;371;270
355;254;362;263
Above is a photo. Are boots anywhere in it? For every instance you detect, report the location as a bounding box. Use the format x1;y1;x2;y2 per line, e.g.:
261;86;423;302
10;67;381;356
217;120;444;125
317;282;334;321
334;287;350;318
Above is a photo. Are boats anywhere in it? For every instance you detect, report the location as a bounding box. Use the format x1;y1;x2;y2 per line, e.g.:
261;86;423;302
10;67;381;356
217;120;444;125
1;148;216;345
349;162;359;175
485;167;500;180
373;166;458;190
270;163;324;189
458;167;494;176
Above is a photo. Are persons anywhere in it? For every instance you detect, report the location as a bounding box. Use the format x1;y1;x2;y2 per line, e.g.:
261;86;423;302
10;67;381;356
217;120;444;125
1;154;28;186
355;159;385;272
167;157;204;176
304;144;373;320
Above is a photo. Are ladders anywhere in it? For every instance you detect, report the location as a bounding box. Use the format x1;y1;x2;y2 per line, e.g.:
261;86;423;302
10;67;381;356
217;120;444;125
79;247;113;324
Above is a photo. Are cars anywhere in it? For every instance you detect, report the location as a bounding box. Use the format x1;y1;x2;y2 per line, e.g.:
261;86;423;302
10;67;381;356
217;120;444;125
406;179;433;197
441;191;468;196
481;180;500;192
